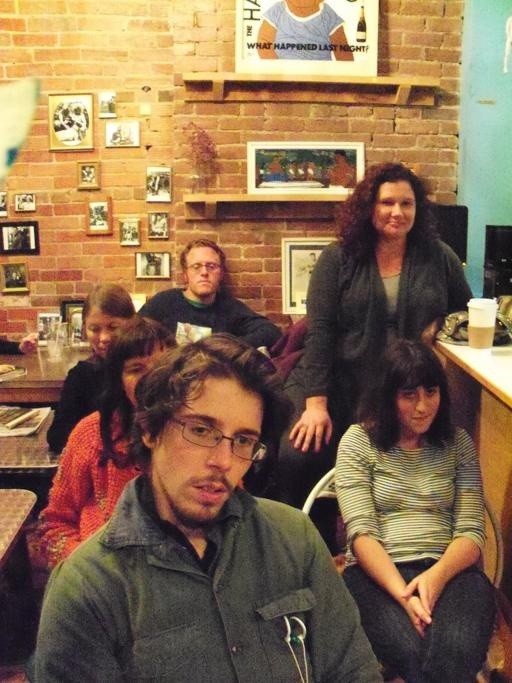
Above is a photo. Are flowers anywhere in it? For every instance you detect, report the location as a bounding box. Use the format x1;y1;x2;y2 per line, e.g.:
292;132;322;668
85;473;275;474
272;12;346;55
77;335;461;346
182;119;224;194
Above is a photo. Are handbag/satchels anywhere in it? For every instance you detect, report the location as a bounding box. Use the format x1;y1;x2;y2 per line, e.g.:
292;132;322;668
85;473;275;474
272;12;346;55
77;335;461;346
436;295;512;345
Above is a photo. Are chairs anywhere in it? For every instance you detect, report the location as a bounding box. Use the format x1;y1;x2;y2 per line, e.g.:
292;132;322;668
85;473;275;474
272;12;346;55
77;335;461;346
302;467;505;683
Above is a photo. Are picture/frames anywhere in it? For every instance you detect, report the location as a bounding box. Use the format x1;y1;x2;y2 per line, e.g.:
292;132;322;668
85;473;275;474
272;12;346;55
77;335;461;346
75;160;103;192
37;292;149;349
235;0;380;78
1;260;31;295
144;165;172;205
102;117;141;149
245;141;369;198
45;91;95;154
117;216;143;248
97;91;119;119
1;219;42;257
133;250;172;280
281;237;341;314
145;211;172;240
82;195;115;238
13;192;37;214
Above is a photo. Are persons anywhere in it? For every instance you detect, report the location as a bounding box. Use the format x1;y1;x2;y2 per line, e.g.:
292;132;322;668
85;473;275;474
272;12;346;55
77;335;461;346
47;282;135;454
33;333;384;682
1;334;38;355
256;0;353;60
266;149;355;187
333;338;497;683
34;314;245;572
135;238;283;347
55;102;87;139
278;160;473;503
5;269;25;287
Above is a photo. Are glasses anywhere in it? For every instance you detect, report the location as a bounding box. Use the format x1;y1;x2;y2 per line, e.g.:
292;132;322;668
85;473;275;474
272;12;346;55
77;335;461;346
169;416;272;463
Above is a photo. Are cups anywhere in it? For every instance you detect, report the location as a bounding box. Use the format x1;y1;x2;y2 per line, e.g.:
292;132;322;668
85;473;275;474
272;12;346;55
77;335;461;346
466;296;500;352
41;320;81;363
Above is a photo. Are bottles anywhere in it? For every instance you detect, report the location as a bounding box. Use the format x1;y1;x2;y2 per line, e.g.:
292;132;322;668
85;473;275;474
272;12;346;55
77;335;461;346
356;5;367;42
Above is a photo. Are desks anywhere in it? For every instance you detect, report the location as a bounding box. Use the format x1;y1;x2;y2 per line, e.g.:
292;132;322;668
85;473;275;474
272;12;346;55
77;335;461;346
0;348;95;403
0;488;38;570
0;406;62;477
435;338;512;595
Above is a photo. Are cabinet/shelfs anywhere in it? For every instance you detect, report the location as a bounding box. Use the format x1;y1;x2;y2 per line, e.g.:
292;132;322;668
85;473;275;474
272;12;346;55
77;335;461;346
182;70;443;223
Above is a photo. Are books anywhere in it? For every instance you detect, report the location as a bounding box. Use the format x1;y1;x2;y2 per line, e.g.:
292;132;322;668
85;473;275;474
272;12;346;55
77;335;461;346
0;363;27;382
1;404;51;438
